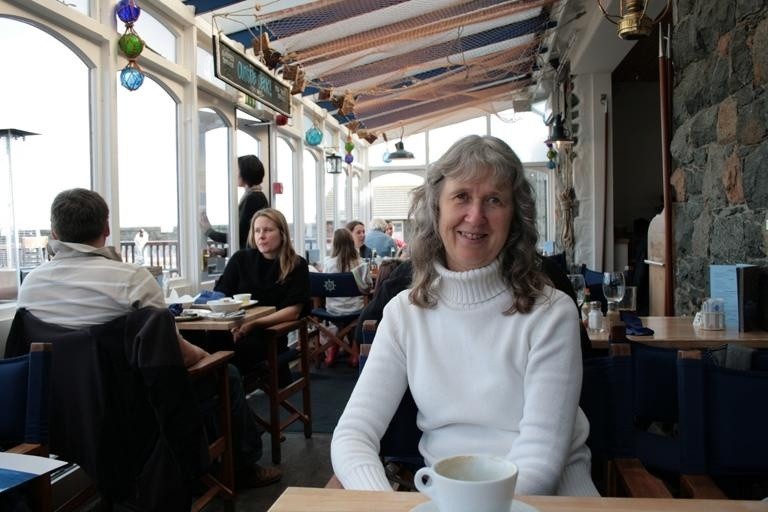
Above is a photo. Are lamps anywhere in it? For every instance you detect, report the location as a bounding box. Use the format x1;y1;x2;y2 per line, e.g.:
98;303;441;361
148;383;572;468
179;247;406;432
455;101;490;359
543;82;574;145
599;1;668;39
388;122;412;159
325;144;343;176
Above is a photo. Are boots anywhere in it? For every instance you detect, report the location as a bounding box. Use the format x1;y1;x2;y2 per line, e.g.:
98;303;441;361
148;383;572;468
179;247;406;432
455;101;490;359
350;341;359;368
324;344;338;368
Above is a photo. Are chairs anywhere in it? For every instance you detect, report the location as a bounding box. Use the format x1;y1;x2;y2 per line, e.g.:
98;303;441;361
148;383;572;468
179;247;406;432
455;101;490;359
241;300;312;466
13;307;233;511
307;272;375;367
680;369;766;499
363;320;626;356
324;344;671;497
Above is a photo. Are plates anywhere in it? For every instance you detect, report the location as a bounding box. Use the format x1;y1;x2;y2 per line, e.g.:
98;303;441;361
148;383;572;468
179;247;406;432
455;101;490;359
241;300;259;308
409;498;538;512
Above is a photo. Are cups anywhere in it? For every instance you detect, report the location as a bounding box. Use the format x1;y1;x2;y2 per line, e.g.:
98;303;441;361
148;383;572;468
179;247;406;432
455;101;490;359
415;456;518;512
233;294;252;302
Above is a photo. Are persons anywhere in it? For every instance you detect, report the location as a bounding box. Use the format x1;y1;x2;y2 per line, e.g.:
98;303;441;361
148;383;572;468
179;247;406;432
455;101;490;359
213;208;315;389
363;216;397;258
346;220;371;259
384;219;405;248
200;154;268;257
322;228;374;366
16;187;283;490
330;133;605;497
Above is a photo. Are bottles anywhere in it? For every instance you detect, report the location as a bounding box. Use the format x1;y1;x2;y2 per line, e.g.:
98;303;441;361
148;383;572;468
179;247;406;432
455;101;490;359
566;274;585;306
589;301;603;332
371;248;379;274
606;301;620;330
602;272;625;303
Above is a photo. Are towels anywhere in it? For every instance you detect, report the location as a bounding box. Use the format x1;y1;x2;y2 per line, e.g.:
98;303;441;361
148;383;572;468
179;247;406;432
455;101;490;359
170;303;181;315
620;311;654;336
195;290;225;304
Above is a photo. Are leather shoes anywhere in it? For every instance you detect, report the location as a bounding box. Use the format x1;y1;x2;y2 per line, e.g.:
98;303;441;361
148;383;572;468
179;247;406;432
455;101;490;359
249;467;282;488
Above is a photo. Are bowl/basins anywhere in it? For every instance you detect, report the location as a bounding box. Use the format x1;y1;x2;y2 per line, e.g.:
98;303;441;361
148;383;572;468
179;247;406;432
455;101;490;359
207;300;243;312
